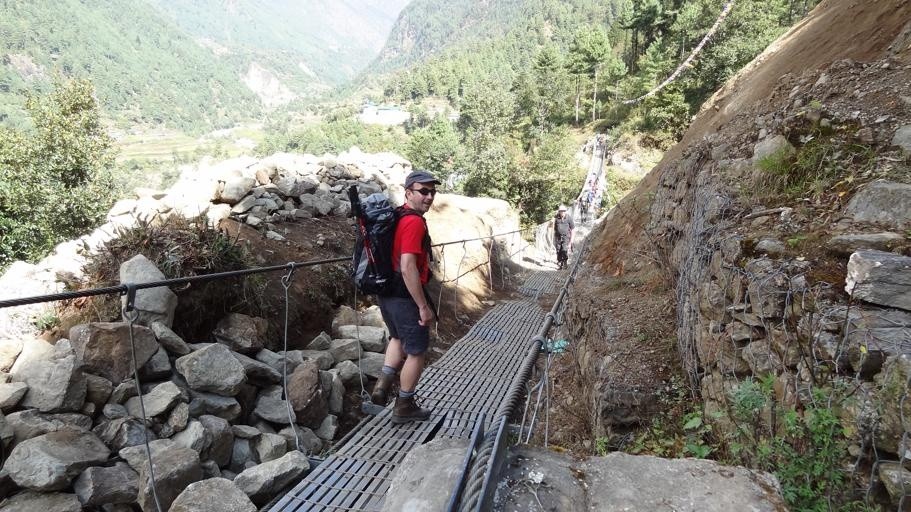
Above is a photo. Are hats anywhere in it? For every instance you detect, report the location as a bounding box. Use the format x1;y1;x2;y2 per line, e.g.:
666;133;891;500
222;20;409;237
405;170;441;188
558;206;566;211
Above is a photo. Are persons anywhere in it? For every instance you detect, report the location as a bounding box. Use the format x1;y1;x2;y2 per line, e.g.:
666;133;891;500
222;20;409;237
577;172;599;224
371;168;440;428
550;205;575;271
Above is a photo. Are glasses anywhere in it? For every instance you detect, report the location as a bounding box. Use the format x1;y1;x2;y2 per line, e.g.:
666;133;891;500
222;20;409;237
405;186;437;196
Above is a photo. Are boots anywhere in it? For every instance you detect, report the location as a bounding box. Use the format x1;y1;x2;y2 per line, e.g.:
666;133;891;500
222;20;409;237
391;394;431;424
557;260;567;269
371;371;399;407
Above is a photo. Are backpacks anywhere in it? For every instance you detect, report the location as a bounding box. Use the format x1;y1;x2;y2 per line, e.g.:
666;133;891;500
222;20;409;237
349;193;428;296
